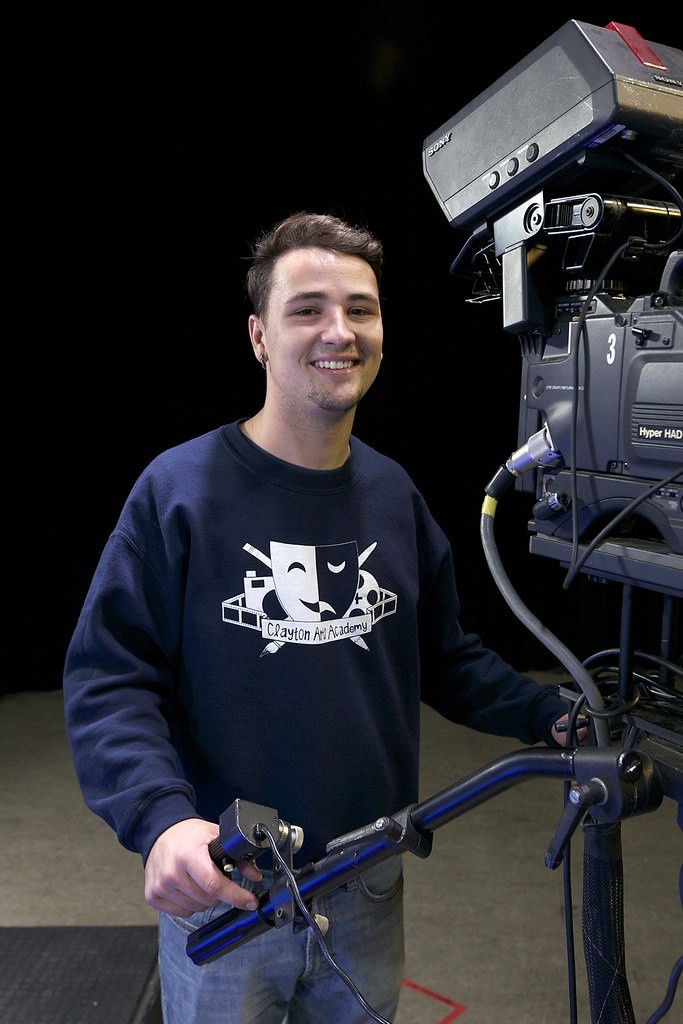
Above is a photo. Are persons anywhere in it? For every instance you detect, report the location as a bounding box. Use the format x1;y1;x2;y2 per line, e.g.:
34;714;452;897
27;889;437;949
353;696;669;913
63;211;590;1024
